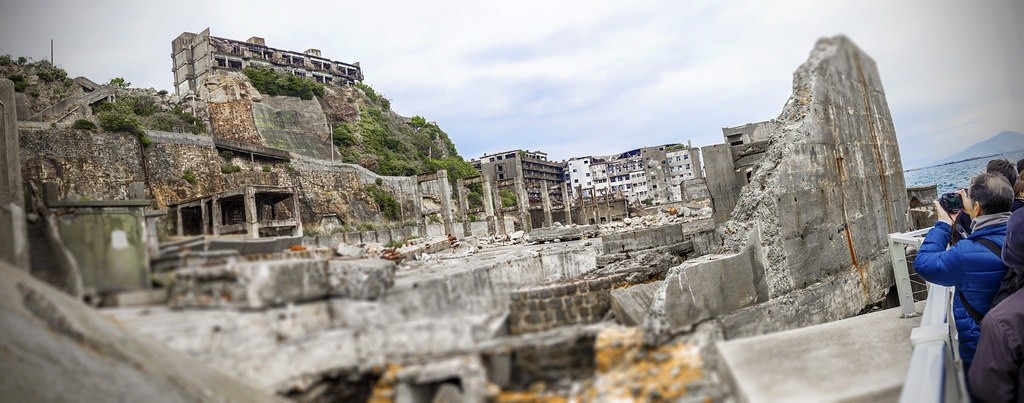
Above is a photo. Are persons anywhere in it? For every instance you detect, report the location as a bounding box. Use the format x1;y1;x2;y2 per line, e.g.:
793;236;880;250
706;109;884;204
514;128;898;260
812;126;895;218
912;159;1024;403
966;207;1024;403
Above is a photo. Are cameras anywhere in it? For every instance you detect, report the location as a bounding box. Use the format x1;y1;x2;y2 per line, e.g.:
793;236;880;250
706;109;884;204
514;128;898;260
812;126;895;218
939;193;963;214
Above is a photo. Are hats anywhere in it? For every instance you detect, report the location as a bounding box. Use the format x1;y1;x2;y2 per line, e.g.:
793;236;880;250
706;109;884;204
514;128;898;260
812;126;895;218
1001;208;1024;273
